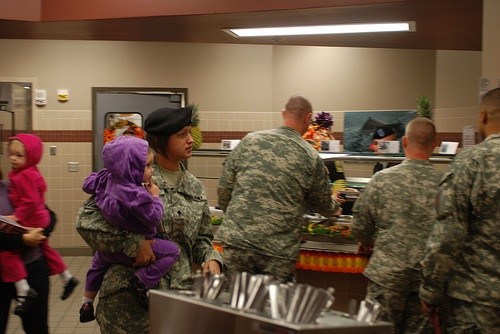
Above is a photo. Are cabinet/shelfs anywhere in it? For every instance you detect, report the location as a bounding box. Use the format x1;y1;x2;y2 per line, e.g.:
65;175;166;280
190;151;452;314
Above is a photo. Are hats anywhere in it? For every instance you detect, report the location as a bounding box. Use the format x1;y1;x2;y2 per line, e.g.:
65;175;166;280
143;102;195;137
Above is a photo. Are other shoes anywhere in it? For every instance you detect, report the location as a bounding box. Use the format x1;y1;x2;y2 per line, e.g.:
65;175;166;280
60;277;78;300
80;301;95;322
13;288;38;315
127;276;149;311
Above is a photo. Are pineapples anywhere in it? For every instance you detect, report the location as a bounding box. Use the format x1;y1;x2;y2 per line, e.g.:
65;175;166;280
190;102;202;150
414;94;432;119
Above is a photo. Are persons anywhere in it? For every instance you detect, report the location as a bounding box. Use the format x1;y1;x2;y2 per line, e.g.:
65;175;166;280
0;131;82;316
0;168;58;334
75;103;225;334
417;86;500;334
215;95;343;284
351;116;448;334
78;134;182;325
